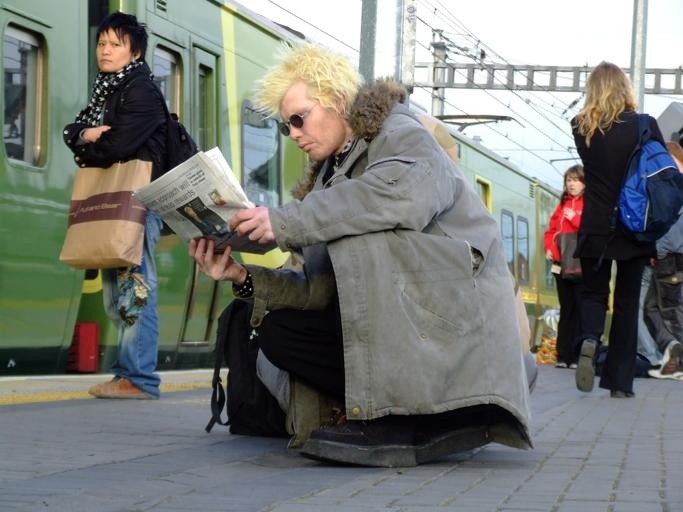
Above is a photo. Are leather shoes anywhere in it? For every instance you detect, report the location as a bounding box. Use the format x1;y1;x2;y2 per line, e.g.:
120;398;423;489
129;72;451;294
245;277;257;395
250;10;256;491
610;389;633;397
576;339;596;393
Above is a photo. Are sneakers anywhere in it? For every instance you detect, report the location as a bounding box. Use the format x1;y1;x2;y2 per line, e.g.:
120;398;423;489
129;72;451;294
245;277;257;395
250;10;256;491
89;376;157;398
647;339;683;379
302;405;490;465
554;360;577;370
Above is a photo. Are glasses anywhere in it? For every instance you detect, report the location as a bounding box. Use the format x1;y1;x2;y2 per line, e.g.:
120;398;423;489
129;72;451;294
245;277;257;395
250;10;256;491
278;102;316;136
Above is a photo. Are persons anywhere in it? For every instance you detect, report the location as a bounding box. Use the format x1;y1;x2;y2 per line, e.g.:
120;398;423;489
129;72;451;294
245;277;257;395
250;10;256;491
185;37;541;472
642;141;683;383
542;164;586;370
63;11;174;404
569;61;671;397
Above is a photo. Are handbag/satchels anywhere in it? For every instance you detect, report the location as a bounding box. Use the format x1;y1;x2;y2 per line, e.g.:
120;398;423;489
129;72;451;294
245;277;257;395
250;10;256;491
616;114;682;242
558;233;582;270
597;343;660;378
59;159;152;269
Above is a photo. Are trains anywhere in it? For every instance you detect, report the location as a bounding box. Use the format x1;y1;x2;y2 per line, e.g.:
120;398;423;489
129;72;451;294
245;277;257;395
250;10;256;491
0;0;614;386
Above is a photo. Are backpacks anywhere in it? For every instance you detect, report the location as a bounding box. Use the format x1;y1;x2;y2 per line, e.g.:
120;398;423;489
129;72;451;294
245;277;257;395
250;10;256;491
206;299;292;436
121;74;197;235
